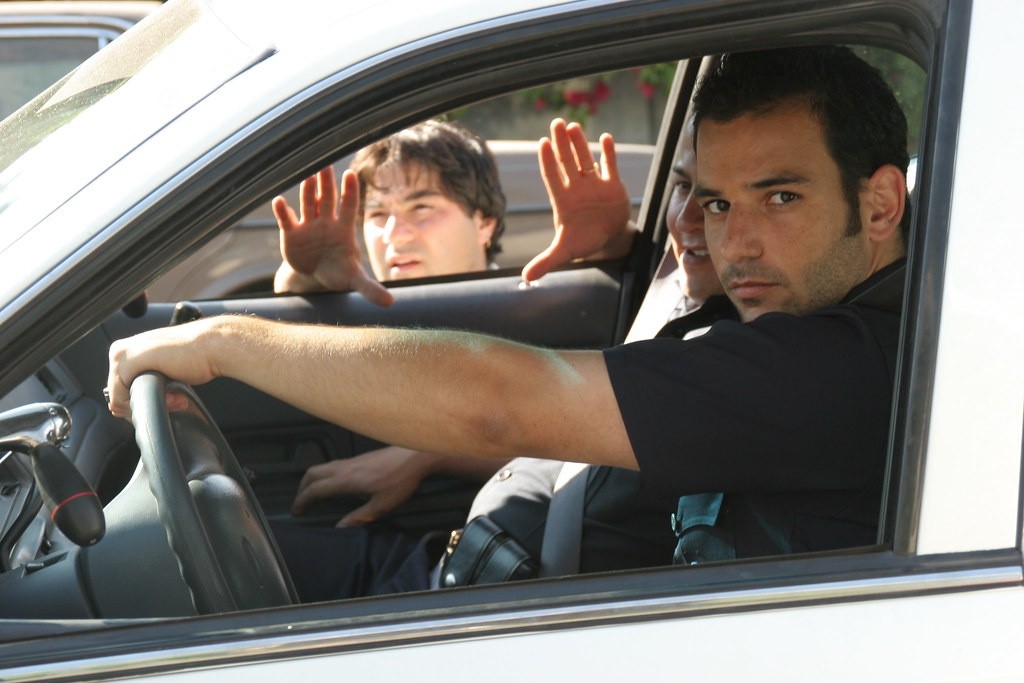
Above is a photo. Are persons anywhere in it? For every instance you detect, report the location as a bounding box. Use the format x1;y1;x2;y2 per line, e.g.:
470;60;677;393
271;118;637;306
103;43;913;563
268;115;724;603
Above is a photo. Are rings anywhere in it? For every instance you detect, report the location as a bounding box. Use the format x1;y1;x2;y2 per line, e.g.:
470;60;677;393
581;164;598;175
103;387;110;403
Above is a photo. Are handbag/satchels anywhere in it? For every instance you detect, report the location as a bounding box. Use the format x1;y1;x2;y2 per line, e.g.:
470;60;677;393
439;513;538;588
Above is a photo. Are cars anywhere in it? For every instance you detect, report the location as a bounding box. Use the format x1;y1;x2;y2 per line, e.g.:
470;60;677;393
0;0;1023;681
